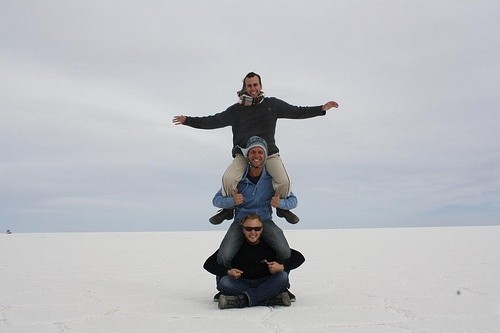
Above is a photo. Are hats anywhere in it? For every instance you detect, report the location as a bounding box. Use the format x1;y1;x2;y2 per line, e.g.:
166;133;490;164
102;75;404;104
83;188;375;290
233;136;268;168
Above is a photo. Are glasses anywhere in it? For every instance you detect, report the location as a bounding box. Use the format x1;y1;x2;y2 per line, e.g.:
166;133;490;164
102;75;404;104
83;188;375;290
242;226;263;232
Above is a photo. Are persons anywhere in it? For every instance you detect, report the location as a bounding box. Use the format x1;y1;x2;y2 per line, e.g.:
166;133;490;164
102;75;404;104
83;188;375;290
202;213;305;309
212;135;298;302
173;71;340;225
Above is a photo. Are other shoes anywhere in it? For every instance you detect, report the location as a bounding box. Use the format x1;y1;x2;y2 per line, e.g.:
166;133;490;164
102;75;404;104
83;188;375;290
214;292;227;302
209;208;234;225
276;207;299;224
267;292;291;307
279;288;295;302
219;295;245;308
